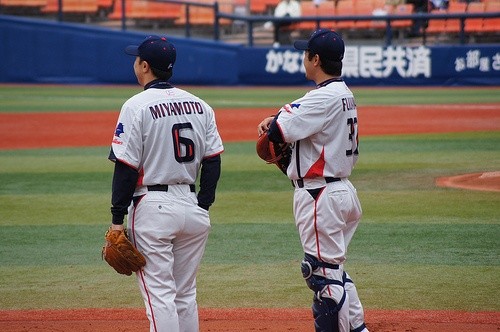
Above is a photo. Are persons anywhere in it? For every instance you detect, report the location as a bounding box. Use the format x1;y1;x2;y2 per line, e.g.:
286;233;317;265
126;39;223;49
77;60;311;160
405;0;441;39
108;37;225;332
257;28;369;332
264;0;301;42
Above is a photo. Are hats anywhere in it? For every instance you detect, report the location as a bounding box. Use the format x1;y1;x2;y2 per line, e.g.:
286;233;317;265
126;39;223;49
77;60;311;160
292;28;346;64
123;31;179;73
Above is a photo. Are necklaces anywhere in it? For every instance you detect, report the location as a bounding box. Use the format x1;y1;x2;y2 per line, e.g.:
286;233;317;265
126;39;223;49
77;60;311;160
149;82;173;90
316;78;344;89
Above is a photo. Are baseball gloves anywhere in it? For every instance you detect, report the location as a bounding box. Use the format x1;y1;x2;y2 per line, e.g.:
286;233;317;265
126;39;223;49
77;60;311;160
256;130;292;176
99;227;147;276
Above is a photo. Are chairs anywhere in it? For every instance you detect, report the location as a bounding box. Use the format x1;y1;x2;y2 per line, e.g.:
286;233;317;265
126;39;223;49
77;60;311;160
0;0;500;44
